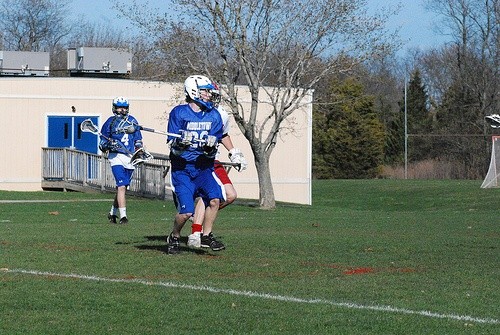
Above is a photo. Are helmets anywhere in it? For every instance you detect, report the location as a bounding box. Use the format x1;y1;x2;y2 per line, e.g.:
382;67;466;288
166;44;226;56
112;96;130;123
183;75;214;112
211;81;220;95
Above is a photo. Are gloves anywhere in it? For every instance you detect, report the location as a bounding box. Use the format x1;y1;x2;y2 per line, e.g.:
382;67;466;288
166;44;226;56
201;134;218;153
99;140;119;151
175;131;195;151
228;148;248;172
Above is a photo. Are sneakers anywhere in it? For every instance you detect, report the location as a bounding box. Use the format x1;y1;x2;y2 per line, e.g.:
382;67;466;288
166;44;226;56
201;232;225;251
167;232;181;255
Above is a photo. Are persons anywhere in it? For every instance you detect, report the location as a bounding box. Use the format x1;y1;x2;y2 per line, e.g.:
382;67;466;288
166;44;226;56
167;75;226;256
99;96;148;224
170;81;247;250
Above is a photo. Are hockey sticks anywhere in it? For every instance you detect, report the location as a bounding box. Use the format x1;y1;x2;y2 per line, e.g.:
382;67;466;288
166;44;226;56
123;122;206;145
80;119;136;155
129;148;239;167
483;113;500;129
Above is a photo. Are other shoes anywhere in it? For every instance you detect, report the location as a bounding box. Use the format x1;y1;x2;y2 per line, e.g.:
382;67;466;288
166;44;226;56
187;231;203;249
119;216;128;224
189;214;194;223
107;214;119;224
